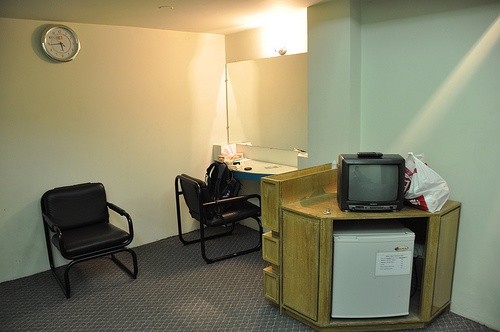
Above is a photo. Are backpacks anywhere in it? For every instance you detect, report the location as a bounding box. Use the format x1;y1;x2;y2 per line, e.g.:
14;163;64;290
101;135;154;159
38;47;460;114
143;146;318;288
205;160;242;215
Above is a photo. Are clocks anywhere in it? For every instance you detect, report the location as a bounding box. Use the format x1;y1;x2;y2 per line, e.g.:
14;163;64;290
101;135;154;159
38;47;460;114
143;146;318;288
41;24;80;62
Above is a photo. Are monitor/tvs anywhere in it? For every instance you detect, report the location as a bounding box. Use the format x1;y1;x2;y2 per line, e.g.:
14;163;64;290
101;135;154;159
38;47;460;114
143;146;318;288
337;152;405;210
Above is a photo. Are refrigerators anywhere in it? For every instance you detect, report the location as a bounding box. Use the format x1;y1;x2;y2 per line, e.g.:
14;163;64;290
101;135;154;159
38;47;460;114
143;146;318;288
330;220;415;318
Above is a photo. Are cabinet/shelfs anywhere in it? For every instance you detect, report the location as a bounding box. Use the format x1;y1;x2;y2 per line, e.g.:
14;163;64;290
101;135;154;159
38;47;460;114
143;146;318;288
279;207;461;332
261;162;337;306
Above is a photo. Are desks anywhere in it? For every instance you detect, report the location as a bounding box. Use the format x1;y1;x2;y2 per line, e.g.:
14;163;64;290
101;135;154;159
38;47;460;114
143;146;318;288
225;158;298;174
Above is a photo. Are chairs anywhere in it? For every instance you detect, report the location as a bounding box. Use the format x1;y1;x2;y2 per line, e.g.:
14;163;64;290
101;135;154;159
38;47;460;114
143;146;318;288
175;174;263;264
40;181;138;298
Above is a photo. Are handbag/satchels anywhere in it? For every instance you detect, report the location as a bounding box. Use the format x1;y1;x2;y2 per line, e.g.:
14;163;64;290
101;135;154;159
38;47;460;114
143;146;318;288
403;151;450;214
